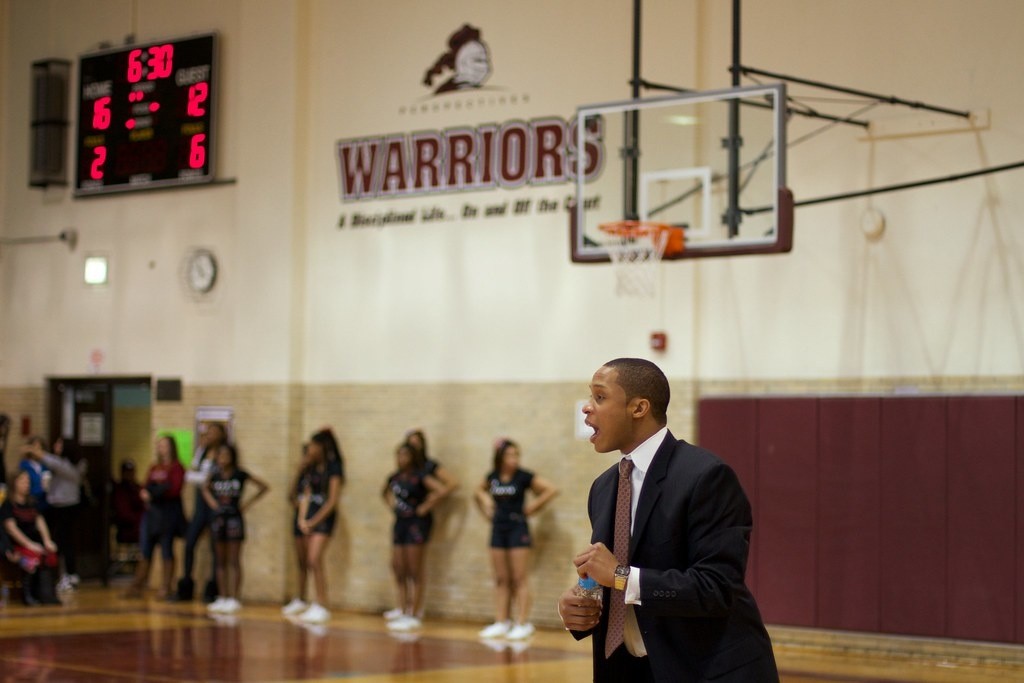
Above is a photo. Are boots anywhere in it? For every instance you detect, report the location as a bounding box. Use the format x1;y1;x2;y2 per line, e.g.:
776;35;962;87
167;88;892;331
200;579;222;603
178;575;195;600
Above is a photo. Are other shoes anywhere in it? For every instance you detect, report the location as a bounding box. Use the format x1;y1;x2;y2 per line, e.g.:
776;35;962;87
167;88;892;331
118;584;142;596
383;608;404;620
151;588;172;599
386;616;420;630
509;622;535;640
208;596;242;613
297;603;332;622
281;597;308;616
478;620;513;637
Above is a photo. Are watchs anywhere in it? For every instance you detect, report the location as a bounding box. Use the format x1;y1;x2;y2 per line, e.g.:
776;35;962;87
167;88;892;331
614;563;631;590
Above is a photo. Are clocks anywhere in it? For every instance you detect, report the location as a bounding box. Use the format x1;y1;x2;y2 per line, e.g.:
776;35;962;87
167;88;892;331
185;250;218;294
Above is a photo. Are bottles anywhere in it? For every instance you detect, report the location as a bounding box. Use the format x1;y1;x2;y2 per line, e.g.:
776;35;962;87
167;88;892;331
576;575;603;609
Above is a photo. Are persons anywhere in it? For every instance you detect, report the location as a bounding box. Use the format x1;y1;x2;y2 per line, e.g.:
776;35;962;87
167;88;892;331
280;426;348;625
0;435;93;606
170;420;270;613
380;431;460;632
558;359;780;683
474;440;561;640
131;434;189;595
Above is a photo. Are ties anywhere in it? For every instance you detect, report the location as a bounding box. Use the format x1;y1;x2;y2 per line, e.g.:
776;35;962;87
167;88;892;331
605;457;635;659
